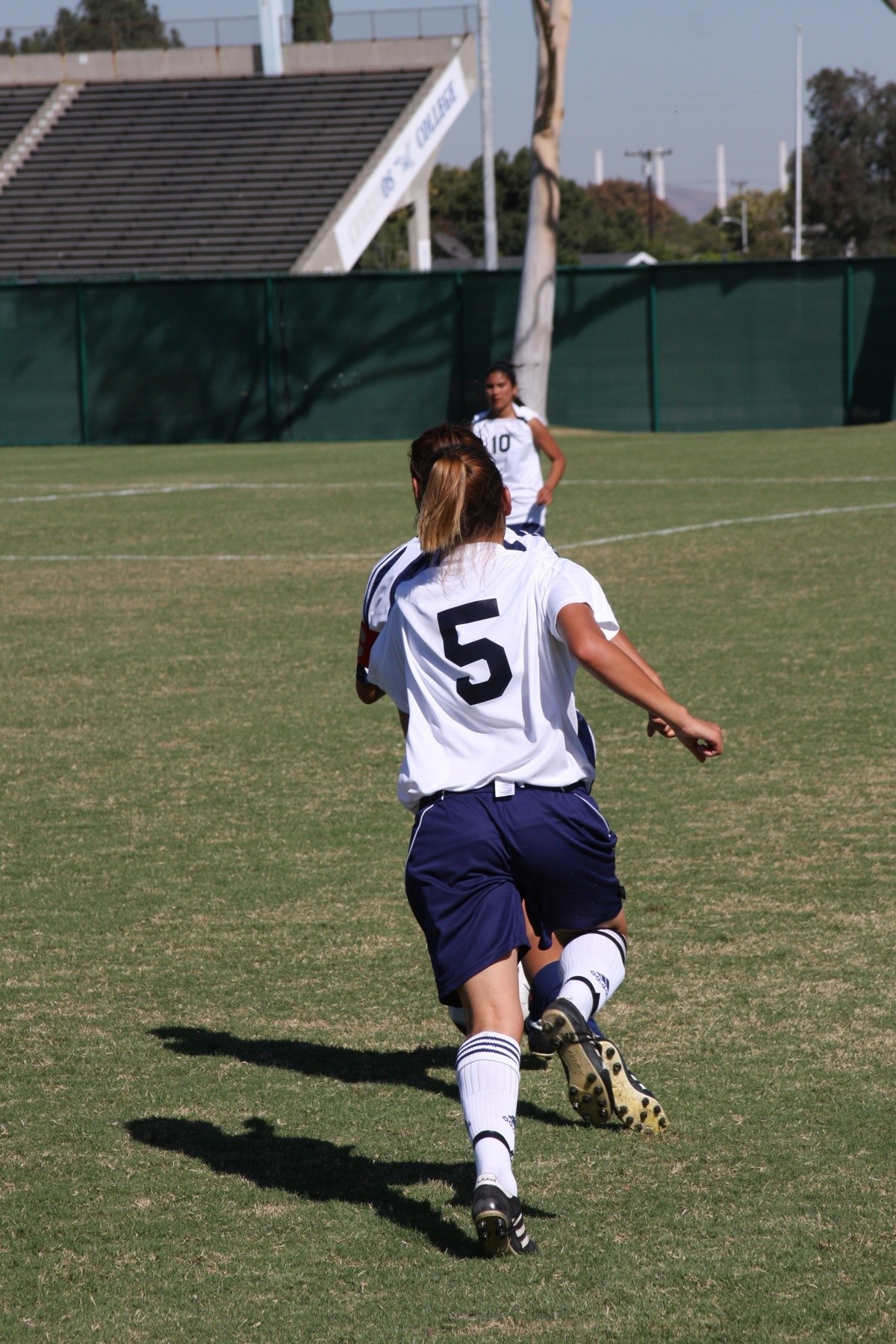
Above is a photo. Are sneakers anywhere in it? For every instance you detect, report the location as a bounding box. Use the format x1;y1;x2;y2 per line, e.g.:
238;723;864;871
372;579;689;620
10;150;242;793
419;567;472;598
537;1000;612;1126
592;1035;670;1136
473;1176;539;1258
525;1016;557;1061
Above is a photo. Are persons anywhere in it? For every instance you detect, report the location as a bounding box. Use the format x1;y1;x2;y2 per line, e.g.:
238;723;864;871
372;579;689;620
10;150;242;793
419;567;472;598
355;423;724;1259
469;361;568;537
